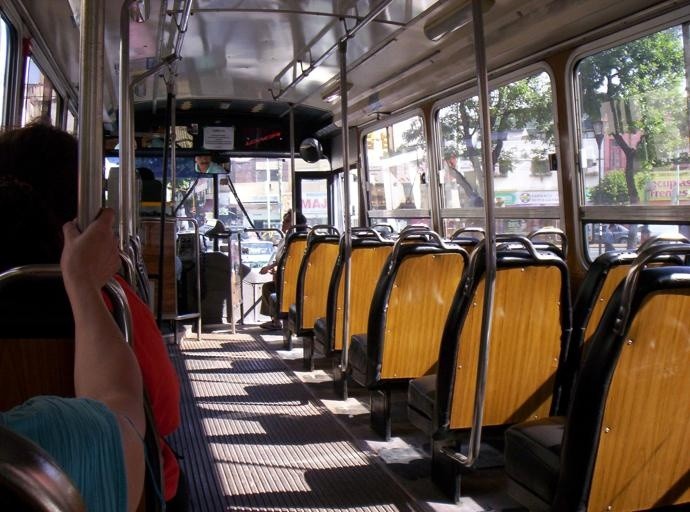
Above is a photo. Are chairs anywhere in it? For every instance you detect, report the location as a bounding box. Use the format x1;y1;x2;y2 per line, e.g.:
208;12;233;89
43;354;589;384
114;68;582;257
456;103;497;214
0;233;156;512
263;217;689;512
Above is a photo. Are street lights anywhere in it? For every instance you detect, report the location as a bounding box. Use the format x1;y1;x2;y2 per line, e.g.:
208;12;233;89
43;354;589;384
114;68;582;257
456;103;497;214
589;118;608;257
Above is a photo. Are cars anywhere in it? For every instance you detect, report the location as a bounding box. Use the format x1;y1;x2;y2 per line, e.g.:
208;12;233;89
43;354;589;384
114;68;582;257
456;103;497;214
588;224;640;245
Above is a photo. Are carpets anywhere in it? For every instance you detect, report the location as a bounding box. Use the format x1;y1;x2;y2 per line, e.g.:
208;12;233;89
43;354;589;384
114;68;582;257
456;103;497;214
155;323;435;512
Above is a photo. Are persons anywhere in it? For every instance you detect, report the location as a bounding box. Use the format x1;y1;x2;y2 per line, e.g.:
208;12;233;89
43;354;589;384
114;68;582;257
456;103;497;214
0;204;148;511
194;155;227;173
641;222;651;245
2;112;194;511
135;165;185;301
602;224;616;252
259;209;308;328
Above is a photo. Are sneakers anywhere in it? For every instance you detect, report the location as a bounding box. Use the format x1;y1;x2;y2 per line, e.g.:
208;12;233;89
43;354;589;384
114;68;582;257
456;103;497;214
260;320;283;329
